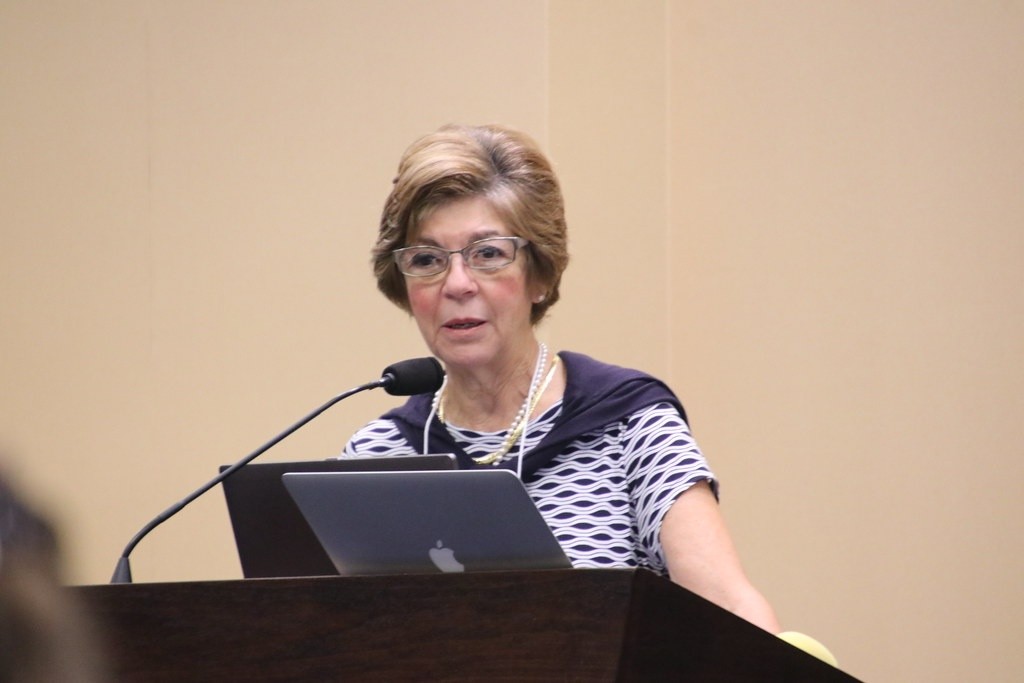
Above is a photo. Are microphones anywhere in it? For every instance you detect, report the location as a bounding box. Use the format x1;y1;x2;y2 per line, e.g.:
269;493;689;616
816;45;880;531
111;356;444;583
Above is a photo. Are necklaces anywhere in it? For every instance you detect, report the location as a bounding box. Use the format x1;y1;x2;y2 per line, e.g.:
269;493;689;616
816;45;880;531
423;342;559;481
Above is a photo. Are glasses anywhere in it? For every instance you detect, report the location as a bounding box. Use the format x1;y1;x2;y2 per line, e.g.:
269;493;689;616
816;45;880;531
392;237;529;277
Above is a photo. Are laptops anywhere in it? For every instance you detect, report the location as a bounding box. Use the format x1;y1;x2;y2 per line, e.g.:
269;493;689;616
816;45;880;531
281;467;572;577
219;453;458;580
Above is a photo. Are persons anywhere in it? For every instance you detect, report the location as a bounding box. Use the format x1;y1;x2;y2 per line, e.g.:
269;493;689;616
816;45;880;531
337;126;780;637
